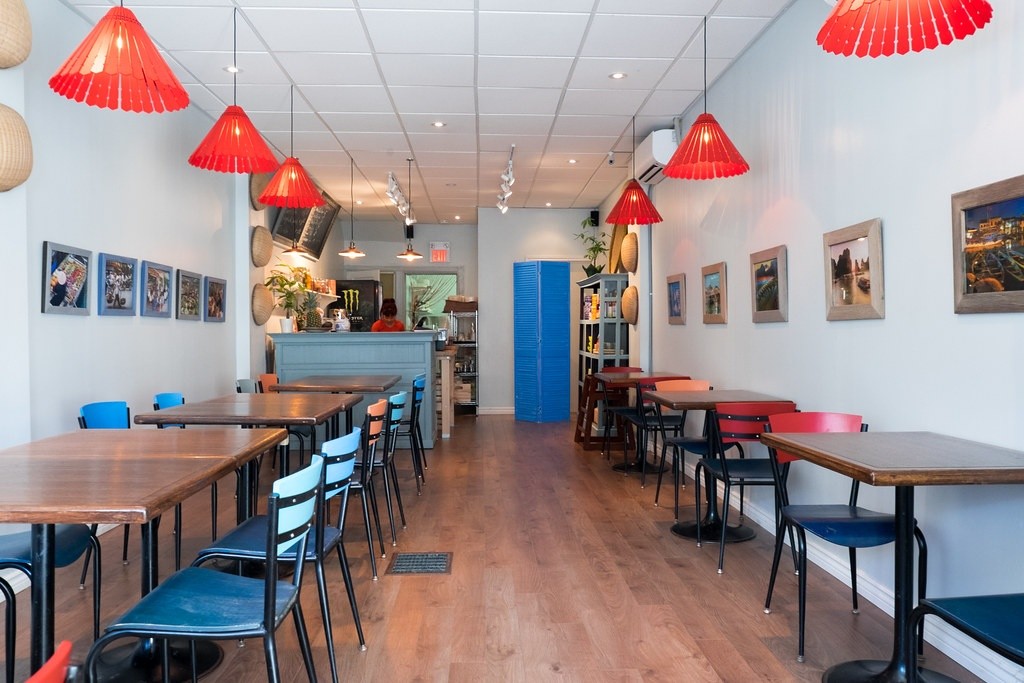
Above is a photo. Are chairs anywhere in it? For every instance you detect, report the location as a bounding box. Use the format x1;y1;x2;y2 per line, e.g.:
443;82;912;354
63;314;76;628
1;368;430;682
598;366;1024;683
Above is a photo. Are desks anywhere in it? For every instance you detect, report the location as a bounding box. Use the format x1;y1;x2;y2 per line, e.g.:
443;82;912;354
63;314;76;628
437;347;455;439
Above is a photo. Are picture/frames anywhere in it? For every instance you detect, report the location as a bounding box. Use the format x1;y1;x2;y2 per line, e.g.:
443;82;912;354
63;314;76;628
664;173;1023;325
41;240;228;325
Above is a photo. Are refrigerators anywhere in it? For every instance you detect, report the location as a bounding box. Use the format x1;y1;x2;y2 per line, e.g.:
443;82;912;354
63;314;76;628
336;277;383;332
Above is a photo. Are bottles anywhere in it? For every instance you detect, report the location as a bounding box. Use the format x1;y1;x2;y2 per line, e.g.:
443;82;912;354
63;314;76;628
470;321;476;342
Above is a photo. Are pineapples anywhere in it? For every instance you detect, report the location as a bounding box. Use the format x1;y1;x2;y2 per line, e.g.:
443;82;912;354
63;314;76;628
304;294;322;328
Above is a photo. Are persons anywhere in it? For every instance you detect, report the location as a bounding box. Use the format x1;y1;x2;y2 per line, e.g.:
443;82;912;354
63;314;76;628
369;298;405;332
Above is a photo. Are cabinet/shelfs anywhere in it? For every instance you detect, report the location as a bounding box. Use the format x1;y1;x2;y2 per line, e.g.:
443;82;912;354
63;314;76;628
574;272;628;434
428;309;479;415
267;331;441;450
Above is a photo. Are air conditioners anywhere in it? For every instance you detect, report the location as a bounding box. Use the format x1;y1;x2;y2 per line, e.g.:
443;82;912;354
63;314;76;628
626;127;678;187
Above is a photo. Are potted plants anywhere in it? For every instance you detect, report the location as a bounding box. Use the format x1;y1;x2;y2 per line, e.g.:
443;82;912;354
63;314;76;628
266;257;302;333
573;214;612;278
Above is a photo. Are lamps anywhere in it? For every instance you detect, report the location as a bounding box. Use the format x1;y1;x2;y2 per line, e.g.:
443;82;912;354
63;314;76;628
256;85;325;209
816;1;994;58
49;0;191;114
188;7;284;171
386;177;418;223
607;118;661;224
398;160;423;261
336;156;366;260
659;17;748;178
495;159;515;214
